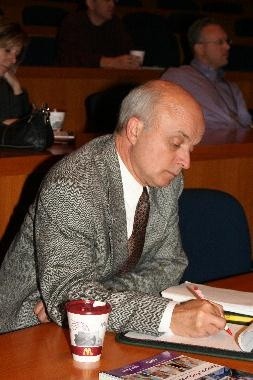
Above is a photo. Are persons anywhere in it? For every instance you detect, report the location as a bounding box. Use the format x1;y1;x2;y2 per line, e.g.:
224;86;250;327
54;0;136;70
0;80;226;338
160;18;253;129
0;16;29;139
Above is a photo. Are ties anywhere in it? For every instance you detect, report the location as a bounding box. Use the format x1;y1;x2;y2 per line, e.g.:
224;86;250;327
123;186;150;274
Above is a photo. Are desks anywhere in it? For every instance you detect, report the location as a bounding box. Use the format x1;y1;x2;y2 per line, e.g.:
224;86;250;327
14;65;253;132
1;270;253;380
23;25;253;47
1;0;253;20
0;128;253;276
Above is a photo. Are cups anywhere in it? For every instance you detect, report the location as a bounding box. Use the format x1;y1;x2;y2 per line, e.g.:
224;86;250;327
50;111;65;131
66;300;112;363
132;51;144;63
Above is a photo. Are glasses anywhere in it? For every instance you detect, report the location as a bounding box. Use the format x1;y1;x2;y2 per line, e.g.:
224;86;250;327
196;38;232;46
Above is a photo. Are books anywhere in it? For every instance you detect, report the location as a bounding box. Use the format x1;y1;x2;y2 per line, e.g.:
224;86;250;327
98;351;253;380
117;281;253;360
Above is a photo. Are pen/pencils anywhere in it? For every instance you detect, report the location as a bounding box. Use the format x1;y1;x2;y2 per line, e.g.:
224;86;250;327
184;281;235;338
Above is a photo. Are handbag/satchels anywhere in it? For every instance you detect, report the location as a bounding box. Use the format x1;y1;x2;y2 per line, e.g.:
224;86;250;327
0;104;55;152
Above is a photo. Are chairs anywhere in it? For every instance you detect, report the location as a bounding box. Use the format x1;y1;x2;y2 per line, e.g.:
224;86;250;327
85;82;137;139
176;187;253;282
22;0;253;71
